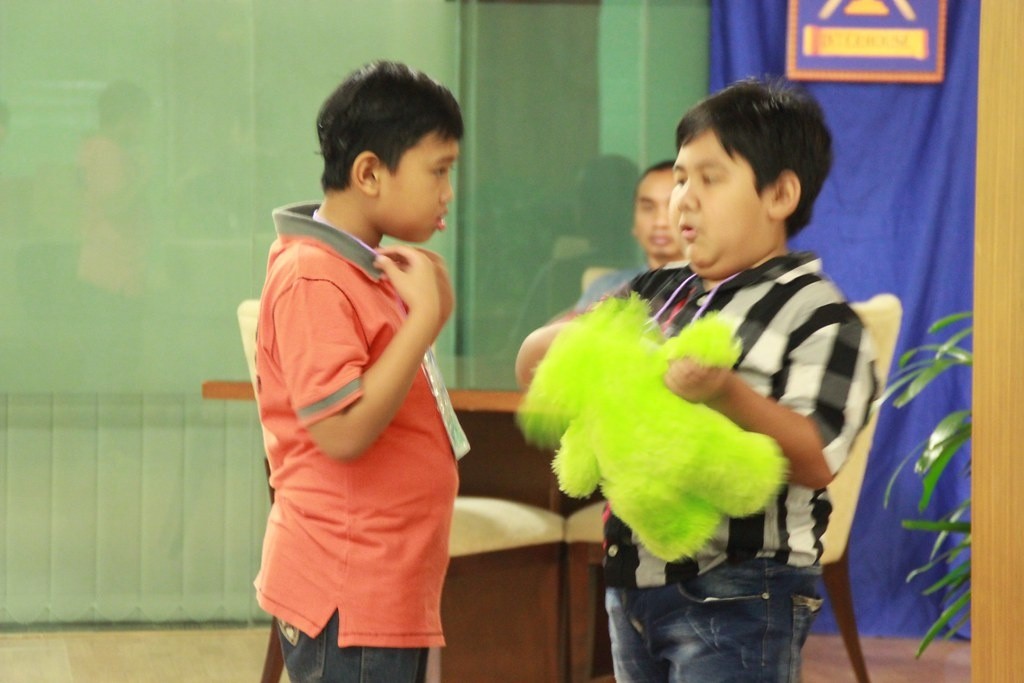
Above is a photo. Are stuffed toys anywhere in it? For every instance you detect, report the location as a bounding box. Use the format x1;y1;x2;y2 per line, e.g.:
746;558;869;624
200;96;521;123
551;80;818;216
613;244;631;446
515;291;787;564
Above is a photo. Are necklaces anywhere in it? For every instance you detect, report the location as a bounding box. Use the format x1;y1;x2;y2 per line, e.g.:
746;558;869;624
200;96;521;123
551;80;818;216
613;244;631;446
649;271;743;329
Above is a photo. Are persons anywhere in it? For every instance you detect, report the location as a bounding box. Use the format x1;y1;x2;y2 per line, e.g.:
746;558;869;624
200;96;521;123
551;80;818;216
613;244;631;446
515;79;878;683
256;58;466;683
551;159;685;327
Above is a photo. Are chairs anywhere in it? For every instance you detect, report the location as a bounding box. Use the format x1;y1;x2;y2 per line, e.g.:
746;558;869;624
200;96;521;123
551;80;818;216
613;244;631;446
237;297;565;682
565;292;906;683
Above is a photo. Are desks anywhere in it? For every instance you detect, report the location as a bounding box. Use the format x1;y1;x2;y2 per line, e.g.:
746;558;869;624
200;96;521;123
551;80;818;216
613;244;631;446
204;380;617;683
